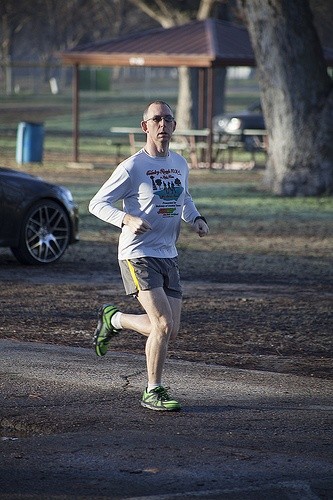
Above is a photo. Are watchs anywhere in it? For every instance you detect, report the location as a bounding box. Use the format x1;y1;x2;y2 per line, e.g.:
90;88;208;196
194;216;207;224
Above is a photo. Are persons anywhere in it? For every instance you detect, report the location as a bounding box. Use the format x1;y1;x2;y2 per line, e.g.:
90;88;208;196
89;101;209;411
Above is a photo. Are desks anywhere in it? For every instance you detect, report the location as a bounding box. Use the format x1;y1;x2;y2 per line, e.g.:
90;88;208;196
211;126;269;172
112;127;209;170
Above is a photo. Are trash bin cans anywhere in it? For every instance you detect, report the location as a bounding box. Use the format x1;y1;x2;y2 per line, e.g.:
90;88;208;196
16;120;44;164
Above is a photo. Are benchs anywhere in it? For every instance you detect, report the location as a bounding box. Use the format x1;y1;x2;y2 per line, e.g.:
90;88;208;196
129;141;208;170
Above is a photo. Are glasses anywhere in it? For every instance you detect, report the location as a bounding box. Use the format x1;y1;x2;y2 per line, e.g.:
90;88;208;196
145;115;174;123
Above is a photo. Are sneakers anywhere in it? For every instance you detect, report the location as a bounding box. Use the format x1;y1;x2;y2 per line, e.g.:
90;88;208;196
140;386;182;410
93;304;122;357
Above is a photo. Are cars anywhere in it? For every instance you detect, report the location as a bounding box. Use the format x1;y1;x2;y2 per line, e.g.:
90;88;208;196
0;166;80;267
213;102;266;141
